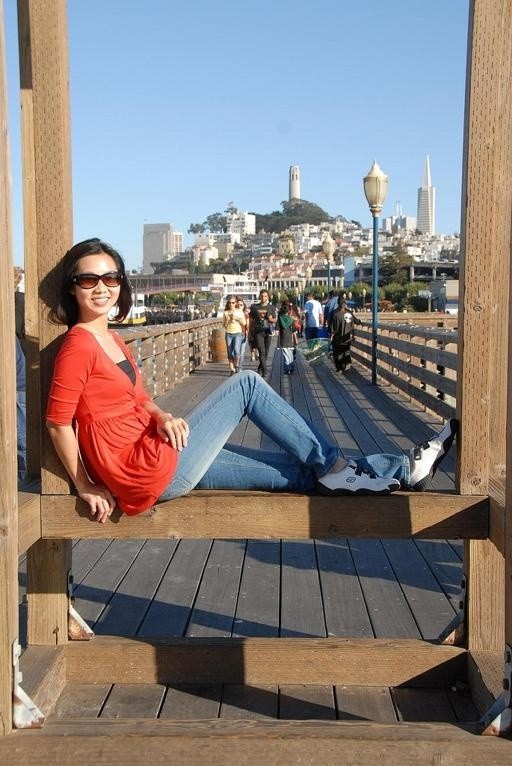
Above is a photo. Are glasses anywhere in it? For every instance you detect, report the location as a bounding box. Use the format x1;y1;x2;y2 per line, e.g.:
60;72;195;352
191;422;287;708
71;271;124;290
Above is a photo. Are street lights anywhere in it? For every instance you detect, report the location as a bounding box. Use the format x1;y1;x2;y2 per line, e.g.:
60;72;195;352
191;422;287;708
427;292;432;310
145;290;198;312
320;229;336;293
292;264;369;312
361;156;392;387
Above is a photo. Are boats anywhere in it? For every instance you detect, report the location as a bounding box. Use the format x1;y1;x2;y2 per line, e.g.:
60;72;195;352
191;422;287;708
217;274;268;317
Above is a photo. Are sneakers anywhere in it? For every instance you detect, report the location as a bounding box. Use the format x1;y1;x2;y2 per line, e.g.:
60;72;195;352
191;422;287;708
397;418;459;492
258;368;267;376
230;366;239;376
315;459;401;497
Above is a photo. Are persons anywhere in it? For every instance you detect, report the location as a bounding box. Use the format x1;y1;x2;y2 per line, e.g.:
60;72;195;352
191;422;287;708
144;303;200;315
44;237;461;523
221;288;371;378
